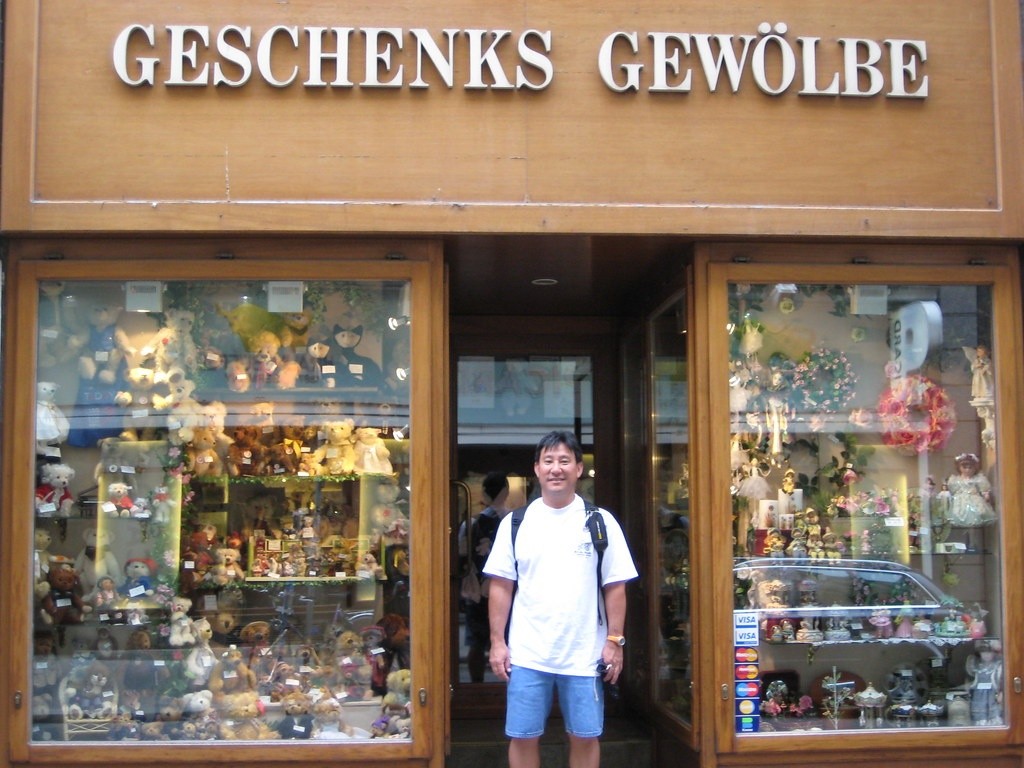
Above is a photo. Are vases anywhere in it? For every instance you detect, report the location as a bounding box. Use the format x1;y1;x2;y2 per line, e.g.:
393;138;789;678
828;518;866;555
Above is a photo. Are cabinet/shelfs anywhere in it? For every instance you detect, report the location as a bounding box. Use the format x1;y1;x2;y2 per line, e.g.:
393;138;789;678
724;280;1011;738
34;278;412;747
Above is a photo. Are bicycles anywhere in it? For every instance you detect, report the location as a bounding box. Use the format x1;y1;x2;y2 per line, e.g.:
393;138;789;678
253;588;377;686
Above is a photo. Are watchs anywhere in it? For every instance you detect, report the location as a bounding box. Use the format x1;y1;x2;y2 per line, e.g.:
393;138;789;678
606;635;625;647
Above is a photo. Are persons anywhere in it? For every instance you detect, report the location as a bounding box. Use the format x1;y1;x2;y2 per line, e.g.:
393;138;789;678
725;342;1006;732
482;430;639;768
458;473;517;685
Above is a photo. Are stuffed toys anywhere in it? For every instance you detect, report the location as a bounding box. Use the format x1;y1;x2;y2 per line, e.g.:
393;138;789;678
31;277;412;747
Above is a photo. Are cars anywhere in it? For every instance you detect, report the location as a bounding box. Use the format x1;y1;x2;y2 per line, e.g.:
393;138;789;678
733;557;984;716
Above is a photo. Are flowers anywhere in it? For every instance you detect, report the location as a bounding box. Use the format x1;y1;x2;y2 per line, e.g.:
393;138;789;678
808;415;827;431
791;345;858;414
841;469;858;486
825;487;899;518
848;407;872;427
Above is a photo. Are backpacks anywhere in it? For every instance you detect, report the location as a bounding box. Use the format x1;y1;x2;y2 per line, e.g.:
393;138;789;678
471;512;503;572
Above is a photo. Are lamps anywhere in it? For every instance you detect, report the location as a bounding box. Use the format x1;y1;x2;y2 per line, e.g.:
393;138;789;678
121;281;166;313
263;281;308;312
848;284;891;314
